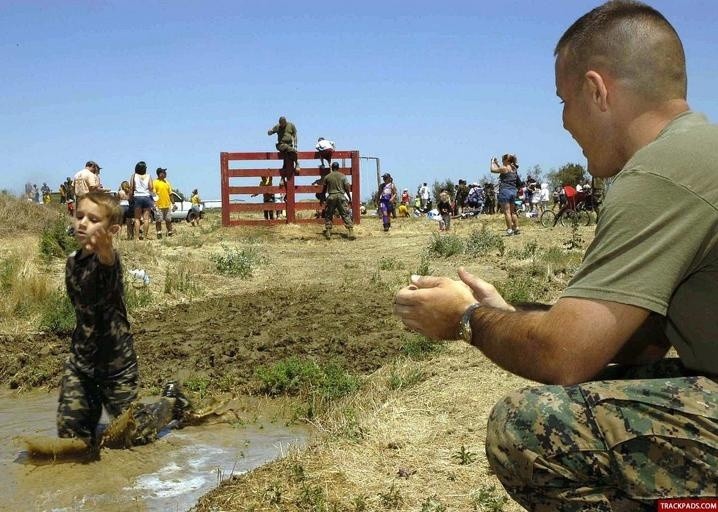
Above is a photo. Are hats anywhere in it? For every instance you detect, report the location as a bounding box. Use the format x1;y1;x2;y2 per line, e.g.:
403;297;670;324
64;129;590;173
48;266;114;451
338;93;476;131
380;173;390;177
156;168;167;174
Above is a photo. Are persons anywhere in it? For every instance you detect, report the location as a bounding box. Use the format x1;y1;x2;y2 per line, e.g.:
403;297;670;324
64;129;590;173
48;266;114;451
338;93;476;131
56;188;197;446
392;6;714;512
22;113;608;246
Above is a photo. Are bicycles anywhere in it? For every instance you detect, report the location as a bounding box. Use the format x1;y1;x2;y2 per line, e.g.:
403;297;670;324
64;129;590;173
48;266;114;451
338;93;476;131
573;200;590;226
539;199;574;228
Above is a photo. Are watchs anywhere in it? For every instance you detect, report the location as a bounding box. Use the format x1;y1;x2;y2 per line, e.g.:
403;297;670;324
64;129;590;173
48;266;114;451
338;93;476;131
455;302;482;345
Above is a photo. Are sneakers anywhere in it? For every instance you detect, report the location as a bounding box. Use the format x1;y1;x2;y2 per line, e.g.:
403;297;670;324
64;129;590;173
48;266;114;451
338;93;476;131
500;229;514;236
163;384;195;417
513;228;520;235
157;234;161;239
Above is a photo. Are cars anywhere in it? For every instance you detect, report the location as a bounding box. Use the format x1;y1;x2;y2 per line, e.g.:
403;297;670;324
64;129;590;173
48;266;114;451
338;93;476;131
120;190;204;221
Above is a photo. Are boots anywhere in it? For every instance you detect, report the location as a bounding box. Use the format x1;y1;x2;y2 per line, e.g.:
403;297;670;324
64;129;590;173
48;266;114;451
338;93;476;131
348;227;356;240
324;229;330;240
